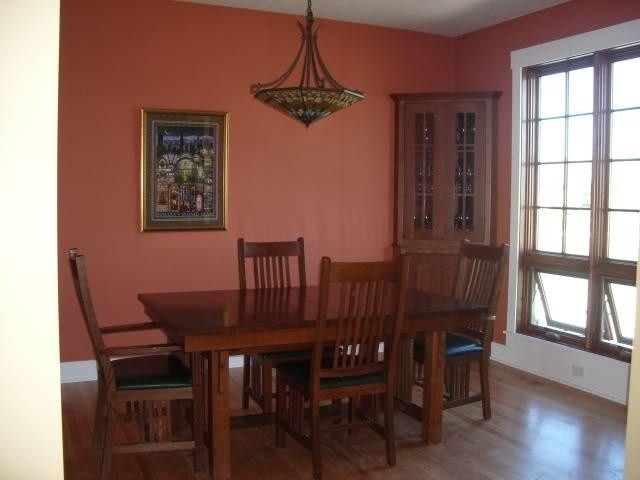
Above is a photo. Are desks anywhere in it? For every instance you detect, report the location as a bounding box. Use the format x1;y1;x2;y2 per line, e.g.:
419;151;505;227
137;279;491;479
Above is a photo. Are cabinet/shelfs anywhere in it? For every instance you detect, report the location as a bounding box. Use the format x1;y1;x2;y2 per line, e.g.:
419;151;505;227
391;90;505;362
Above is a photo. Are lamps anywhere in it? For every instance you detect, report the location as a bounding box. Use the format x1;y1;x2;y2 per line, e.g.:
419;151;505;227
249;2;365;128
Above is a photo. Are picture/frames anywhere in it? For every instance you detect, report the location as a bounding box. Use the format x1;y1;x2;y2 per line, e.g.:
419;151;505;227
138;107;230;231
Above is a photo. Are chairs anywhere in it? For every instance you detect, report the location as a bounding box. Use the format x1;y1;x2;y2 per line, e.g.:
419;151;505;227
235;235;342;415
68;246;208;479
275;253;400;477
392;239;509;421
